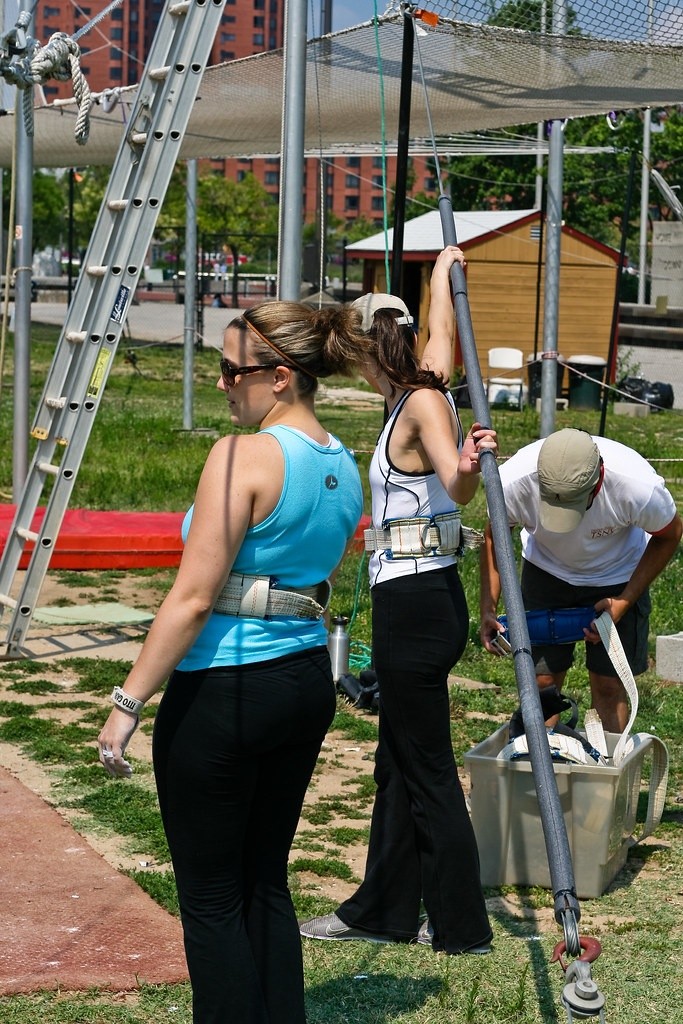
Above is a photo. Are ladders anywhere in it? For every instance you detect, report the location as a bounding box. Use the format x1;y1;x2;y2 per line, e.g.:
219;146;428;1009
0;1;229;663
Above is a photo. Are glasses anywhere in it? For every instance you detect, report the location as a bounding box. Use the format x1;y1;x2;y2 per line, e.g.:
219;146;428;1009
219;358;299;388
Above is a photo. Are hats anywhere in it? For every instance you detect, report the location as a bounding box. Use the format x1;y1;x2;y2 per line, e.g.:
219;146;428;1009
347;292;414;335
537;427;600;534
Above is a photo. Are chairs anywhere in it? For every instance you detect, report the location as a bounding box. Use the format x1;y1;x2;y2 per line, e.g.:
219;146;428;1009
486;347;524;411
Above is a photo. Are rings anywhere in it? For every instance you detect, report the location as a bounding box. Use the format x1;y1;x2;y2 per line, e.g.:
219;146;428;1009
102;750;114;758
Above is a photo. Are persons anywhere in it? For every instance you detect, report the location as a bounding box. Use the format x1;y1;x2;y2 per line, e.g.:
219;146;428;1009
96;299;371;1024
296;246;500;956
482;429;683;733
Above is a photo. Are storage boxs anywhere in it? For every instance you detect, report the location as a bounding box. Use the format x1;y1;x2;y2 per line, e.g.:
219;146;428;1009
464;723;652;899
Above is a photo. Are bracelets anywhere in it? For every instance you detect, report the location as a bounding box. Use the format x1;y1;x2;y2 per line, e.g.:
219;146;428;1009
111;684;144;714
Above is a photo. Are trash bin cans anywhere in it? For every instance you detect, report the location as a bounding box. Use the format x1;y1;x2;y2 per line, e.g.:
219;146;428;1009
567;355;607;410
526;352;566;409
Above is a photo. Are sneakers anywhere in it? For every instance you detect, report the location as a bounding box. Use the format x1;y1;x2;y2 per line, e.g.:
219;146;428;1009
417;918;491;954
298;913;398;944
